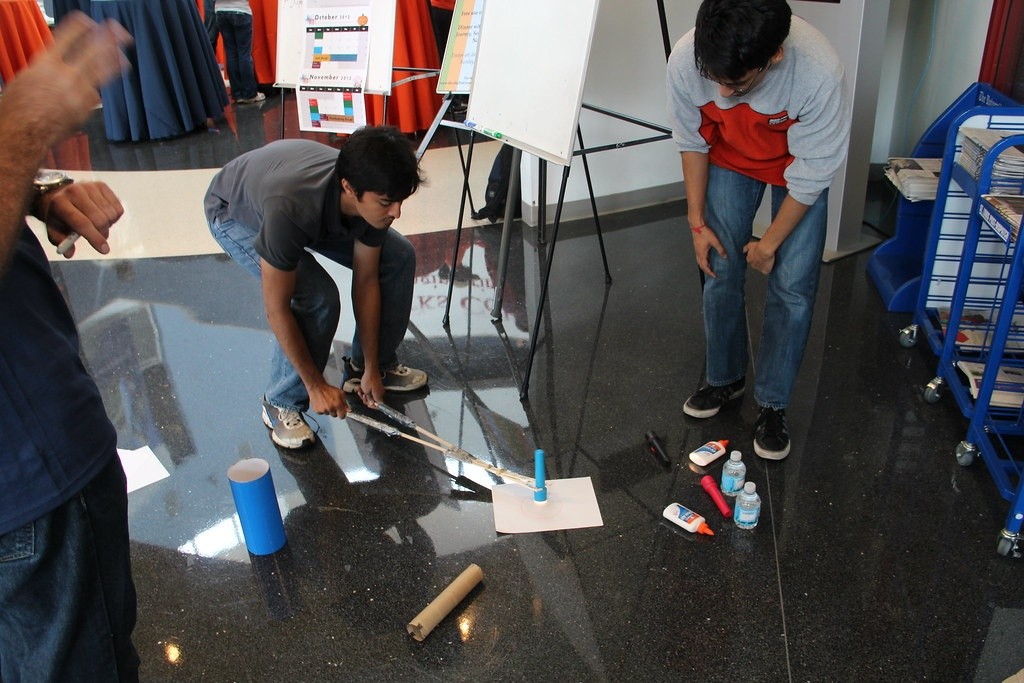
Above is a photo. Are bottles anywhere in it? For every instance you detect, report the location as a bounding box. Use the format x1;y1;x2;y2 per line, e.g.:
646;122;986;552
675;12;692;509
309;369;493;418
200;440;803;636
663;502;714;536
734;482;760;529
720;451;746;497
689;440;729;466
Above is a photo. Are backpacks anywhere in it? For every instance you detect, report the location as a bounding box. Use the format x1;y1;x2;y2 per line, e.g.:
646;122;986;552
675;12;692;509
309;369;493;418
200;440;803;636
471;143;522;223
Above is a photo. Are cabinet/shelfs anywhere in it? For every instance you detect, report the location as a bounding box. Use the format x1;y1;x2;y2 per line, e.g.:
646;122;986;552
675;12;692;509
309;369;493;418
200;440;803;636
896;107;1024;558
867;82;1024;313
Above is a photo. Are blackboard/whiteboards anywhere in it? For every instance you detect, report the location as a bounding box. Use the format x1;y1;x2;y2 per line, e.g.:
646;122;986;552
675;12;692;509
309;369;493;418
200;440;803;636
272;0;396;96
462;1;599;167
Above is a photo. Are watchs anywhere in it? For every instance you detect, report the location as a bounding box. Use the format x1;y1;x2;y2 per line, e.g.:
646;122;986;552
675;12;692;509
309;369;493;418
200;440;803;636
34;171;73;219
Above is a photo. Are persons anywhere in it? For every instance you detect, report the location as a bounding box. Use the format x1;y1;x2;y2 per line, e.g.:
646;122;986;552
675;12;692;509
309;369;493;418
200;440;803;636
215;0;266;103
666;0;849;460
204;125;428;448
0;11;140;683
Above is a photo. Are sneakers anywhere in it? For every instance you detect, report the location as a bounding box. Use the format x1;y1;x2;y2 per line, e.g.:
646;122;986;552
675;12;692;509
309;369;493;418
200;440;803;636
753;407;790;460
339;355;428;393
261;394;320;449
236;91;266;104
683;376;746;418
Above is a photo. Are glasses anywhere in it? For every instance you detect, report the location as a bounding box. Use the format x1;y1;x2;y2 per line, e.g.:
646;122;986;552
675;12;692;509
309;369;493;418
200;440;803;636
699;61;767;95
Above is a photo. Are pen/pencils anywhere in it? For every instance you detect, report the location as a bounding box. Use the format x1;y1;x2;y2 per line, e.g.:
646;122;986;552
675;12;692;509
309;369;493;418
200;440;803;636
56;232;81;254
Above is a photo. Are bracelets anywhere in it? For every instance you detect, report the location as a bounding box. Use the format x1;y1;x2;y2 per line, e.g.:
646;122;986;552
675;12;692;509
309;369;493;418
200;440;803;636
690;223;706;234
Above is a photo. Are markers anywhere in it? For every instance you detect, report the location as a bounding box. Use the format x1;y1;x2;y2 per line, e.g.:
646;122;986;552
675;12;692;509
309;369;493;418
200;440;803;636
208;128;219;133
463;120;479;130
481;127;503;139
